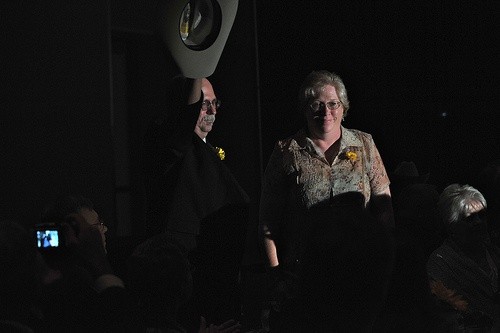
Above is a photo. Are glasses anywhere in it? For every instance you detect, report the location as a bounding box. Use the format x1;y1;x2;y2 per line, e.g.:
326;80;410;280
78;217;105;232
201;98;221;110
307;99;343;111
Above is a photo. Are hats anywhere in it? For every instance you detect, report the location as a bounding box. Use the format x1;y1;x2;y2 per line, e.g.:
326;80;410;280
158;0;239;78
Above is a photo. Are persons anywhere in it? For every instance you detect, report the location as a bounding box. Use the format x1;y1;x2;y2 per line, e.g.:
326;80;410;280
258;70;395;333
0;184;500;333
145;73;251;333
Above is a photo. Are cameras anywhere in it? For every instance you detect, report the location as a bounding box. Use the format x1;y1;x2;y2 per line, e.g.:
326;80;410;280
36;229;61;248
